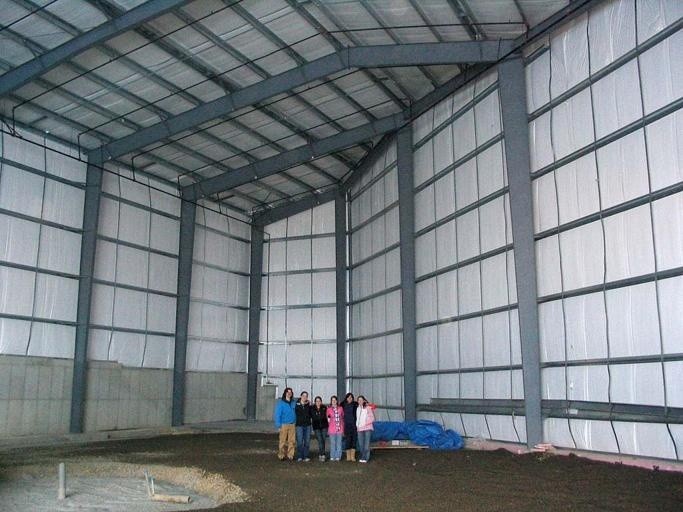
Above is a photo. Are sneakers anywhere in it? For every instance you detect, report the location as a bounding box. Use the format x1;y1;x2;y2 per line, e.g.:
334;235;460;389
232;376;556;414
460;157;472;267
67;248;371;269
279;448;367;464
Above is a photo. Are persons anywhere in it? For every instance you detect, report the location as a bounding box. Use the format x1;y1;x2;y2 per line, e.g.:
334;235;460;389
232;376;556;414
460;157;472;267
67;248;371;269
355;395;375;463
326;396;344;461
295;391;312;462
311;396;329;462
339;393;372;462
273;388;298;461
